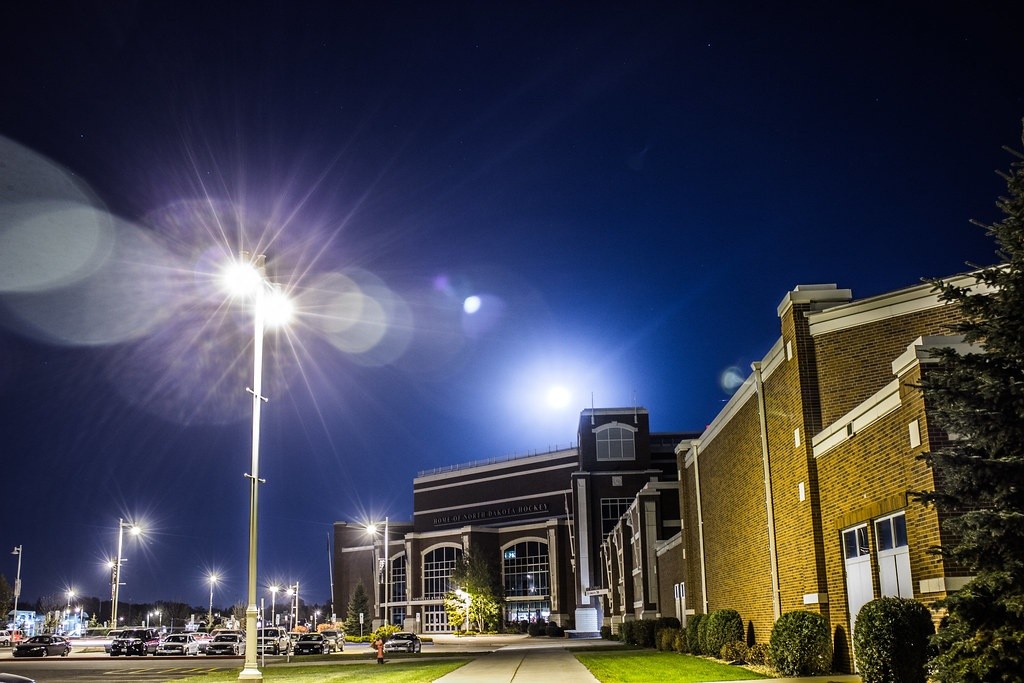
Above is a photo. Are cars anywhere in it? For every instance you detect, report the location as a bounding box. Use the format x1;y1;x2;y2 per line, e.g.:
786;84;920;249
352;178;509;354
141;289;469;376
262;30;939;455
12;634;72;657
206;634;245;655
104;630;125;652
286;631;304;651
156;634;199;656
0;629;26;647
294;633;330;654
110;629;159;656
258;628;292;656
320;630;346;653
383;632;422;654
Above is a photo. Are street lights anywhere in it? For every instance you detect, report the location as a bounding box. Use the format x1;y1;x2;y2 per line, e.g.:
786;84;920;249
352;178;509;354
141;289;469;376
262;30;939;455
156;609;163;628
208;575;217;626
146;611;153;628
456;588;472;633
315;609;321;632
215;248;297;683
269;585;279;626
11;545;23;631
108;517;142;630
368;517;390;628
288;582;299;632
64;588;84;629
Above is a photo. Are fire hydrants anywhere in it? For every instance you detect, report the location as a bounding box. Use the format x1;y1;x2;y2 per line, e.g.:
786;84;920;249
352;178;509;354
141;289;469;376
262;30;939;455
375;639;384;664
